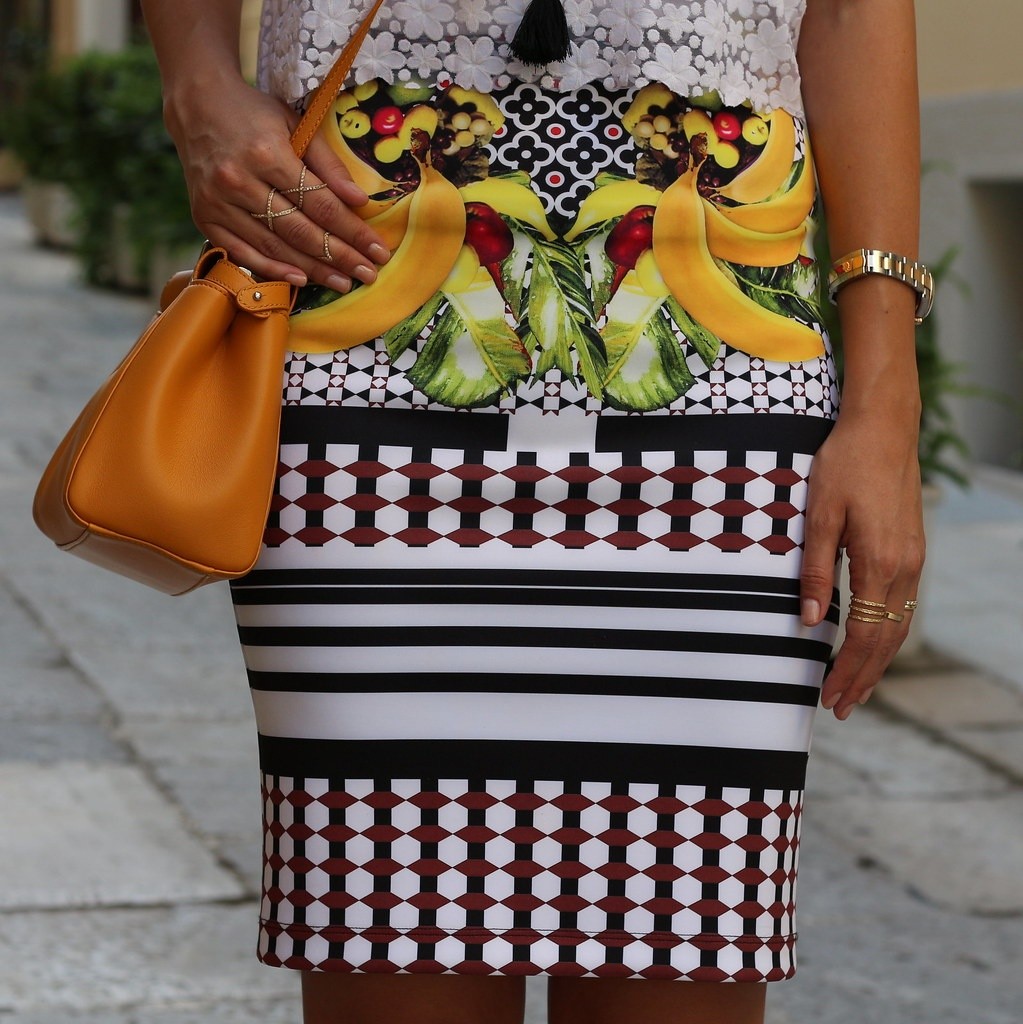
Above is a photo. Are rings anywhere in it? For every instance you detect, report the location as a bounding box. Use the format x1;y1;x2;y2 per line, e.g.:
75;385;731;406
316;233;334;263
250;190;295;233
848;596;886;624
904;600;919;610
281;165;328;209
885;610;904;622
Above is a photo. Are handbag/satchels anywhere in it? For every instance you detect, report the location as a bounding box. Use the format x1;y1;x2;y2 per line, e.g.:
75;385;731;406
31;249;301;600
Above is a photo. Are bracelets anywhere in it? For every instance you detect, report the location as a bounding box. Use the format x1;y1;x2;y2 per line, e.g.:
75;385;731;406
826;248;935;326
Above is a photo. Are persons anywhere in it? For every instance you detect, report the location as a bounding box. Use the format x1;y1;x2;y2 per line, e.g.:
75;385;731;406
144;1;922;1024
